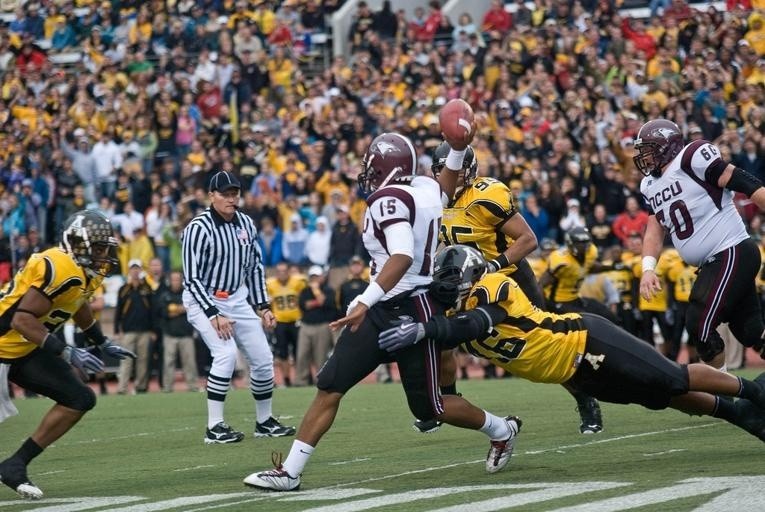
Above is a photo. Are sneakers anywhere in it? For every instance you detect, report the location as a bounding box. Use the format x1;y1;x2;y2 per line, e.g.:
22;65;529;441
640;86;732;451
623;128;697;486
242;451;303;492
486;414;522;474
574;398;603;435
412;418;443;433
0;453;43;499
254;416;296;438
204;421;244;444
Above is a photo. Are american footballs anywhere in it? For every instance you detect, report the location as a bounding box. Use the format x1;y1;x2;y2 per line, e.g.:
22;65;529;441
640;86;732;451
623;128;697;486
441;97;474;142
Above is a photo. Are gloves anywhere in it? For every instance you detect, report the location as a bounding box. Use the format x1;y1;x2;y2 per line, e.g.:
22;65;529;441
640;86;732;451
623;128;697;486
99;335;138;359
378;319;426;352
63;345;104;381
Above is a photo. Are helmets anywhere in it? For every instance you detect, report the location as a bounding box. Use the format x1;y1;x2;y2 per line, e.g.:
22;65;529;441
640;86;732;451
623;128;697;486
539;237;558;251
358;133;417;194
633;119;685;177
564;226;592;256
349;255;364;265
58;209;120;278
431;140;478;186
429;244;488;304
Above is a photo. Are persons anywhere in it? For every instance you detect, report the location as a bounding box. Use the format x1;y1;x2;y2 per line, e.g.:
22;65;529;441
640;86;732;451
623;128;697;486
0;210;136;500
1;0;765;387
412;137;603;437
181;170;297;442
632;119;765;374
377;243;765;444
241;131;524;492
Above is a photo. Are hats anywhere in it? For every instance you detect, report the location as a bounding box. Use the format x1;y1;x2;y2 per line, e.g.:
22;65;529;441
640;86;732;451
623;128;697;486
567;198;580;208
208;170;241;192
336;204;349;213
128;260;141;268
308;265;323;278
